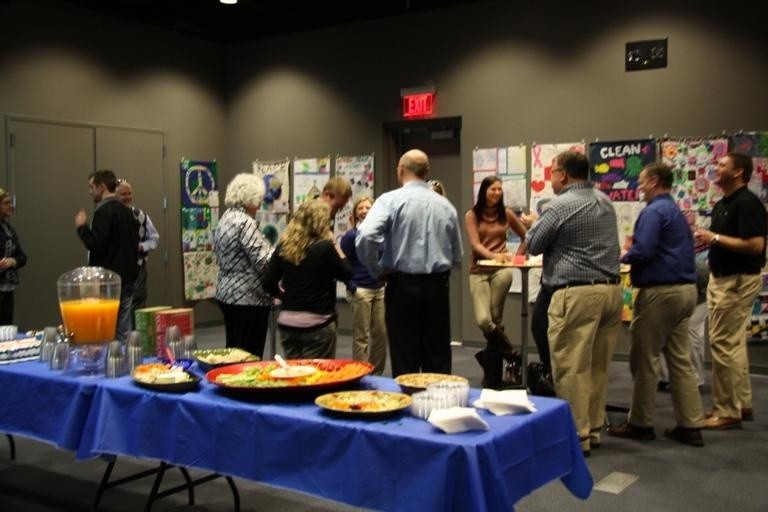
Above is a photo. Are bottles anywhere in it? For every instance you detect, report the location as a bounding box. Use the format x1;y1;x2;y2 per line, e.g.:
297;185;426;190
51;339;66;369
126;331;142;371
166;325;185;359
40;327;56;361
107;341;124;377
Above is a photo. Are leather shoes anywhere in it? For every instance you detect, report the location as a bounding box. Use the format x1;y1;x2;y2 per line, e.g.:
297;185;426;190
605;420;656;440
666;426;703;447
707;407;753;420
702;412;742;430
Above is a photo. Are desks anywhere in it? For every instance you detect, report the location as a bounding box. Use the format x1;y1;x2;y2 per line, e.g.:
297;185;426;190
475;254;629;414
98;359;567;512
1;334;195;511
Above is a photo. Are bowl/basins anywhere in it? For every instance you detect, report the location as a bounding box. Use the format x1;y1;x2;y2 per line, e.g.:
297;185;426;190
192;349;260;371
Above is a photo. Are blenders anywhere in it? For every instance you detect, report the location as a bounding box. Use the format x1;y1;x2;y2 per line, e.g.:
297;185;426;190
55;264;122;374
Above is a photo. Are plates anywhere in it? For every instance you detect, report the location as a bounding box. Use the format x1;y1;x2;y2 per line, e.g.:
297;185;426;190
207;358;374;389
395;373;468;393
315;390;411;413
135;371;201;389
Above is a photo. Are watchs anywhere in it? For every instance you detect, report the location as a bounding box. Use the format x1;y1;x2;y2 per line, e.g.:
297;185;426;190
710;234;720;247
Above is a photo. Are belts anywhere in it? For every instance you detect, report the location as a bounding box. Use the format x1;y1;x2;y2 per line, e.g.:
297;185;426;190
556;280;621;289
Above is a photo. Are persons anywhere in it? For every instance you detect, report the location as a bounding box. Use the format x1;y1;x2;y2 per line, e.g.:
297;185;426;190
606;160;706;447
74;169;140;342
521;151;623;457
692;152;768;430
215;172;276;361
317;175;353;236
520;211;554;387
426;179;447;198
267;200;354;358
340;195;389;376
87;181;160;331
354;148;465;379
0;187;27;325
463;175;528;390
655;269;708;395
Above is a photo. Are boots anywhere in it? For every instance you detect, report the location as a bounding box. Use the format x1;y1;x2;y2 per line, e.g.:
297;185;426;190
482;326;504;389
484;326;523;367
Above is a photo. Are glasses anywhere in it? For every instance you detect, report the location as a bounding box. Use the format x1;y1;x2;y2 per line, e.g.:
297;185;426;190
427;179;440;193
